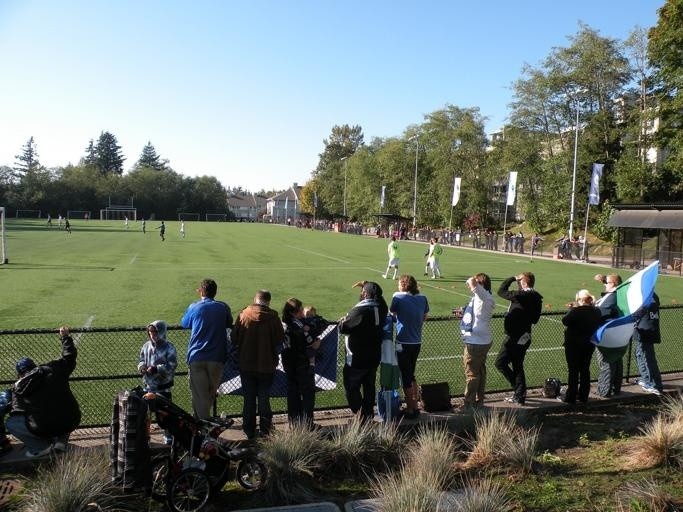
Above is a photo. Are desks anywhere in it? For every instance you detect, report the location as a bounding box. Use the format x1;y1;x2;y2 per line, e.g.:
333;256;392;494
674;257;683;271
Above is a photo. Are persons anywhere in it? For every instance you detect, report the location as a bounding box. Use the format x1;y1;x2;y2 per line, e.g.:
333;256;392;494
5;327;81;459
286;217;363;235
338;281;388;424
451;273;496;414
390;274;429;419
381;236;400;279
376;223;583;259
155;218;184;241
233;290;284;440
594;274;664;404
495;272;542;404
138;320;176;445
281;298;327;428
560;289;602;403
47;213;71;234
181;279;233;424
125;216;145;234
424;238;443;280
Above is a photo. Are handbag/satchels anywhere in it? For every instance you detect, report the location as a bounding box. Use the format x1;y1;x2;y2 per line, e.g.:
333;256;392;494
542;377;560;399
418;380;450;413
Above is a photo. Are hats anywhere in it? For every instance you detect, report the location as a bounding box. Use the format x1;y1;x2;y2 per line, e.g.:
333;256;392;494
14;358;34;372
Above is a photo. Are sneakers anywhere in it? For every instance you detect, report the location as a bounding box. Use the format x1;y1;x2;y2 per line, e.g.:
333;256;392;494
504;394;525;406
25;442;68;458
451;404;465;414
401;407;419;418
638;379;662;397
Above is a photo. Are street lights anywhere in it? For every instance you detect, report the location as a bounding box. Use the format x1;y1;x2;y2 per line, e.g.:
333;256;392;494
408;132;421;230
563;87;590;241
341;157;348;217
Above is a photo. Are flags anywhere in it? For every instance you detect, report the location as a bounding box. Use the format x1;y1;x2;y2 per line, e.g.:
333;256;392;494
505;171;518;206
313;192;318;208
380;186;386;209
452;177;461;207
588;163;605;206
592;263;658;349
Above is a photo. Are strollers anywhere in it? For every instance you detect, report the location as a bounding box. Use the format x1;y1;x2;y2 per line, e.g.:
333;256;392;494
128;386;267;512
